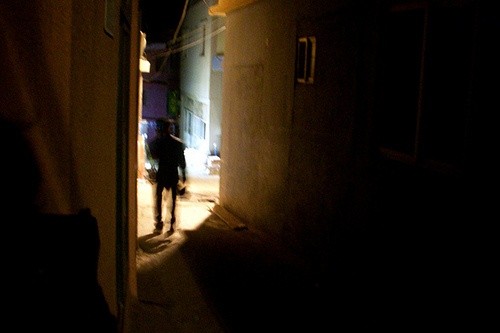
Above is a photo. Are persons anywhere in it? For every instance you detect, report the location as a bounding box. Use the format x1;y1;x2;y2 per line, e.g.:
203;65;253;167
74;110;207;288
147;117;186;235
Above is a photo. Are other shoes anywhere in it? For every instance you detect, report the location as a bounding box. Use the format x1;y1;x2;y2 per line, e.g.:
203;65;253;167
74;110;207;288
168;227;176;234
154;225;164;233
